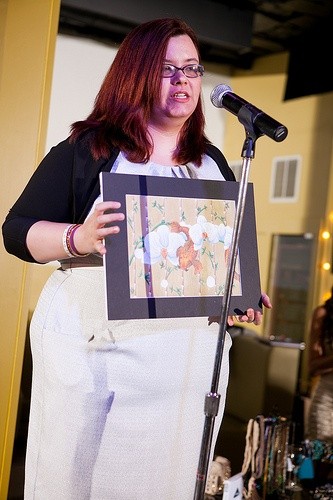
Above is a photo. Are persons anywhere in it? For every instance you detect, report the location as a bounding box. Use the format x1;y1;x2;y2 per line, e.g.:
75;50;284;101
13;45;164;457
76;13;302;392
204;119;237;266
293;283;333;447
0;13;276;500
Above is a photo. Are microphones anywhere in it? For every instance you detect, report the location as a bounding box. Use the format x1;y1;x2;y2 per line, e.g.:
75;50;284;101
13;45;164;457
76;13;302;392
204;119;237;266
210;83;288;142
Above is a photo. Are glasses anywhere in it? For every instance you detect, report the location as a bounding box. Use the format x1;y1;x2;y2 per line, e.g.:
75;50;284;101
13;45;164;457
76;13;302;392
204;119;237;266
162;63;205;78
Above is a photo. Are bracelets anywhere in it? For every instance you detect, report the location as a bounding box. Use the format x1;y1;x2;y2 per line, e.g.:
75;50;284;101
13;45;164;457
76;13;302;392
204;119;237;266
60;223;96;259
